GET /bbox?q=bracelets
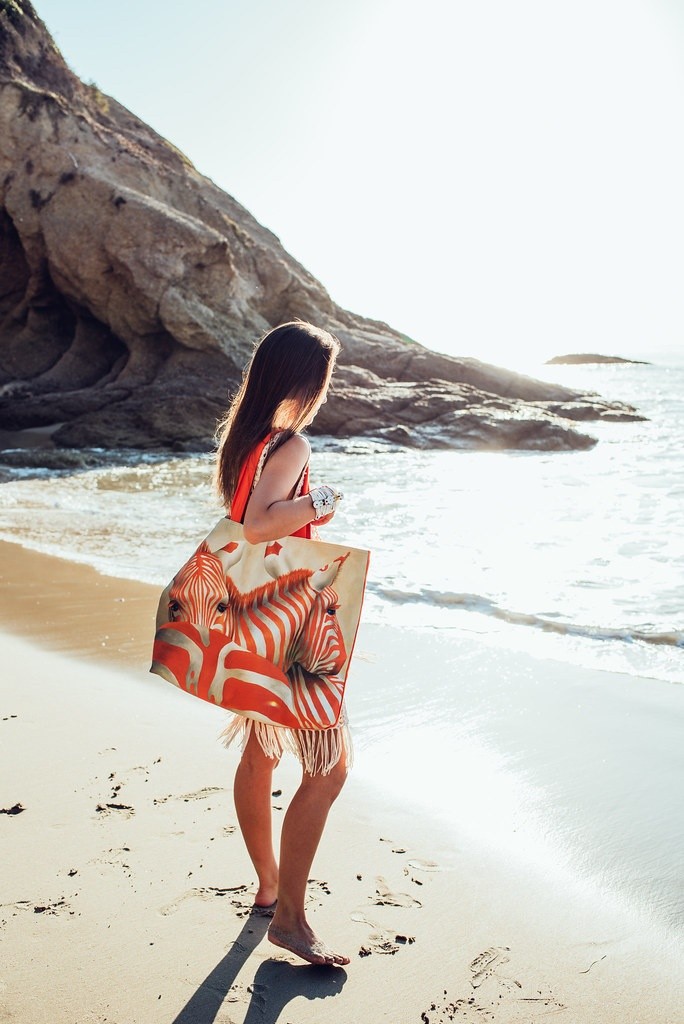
[311,484,344,523]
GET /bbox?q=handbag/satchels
[148,429,372,779]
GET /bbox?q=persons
[209,320,350,966]
